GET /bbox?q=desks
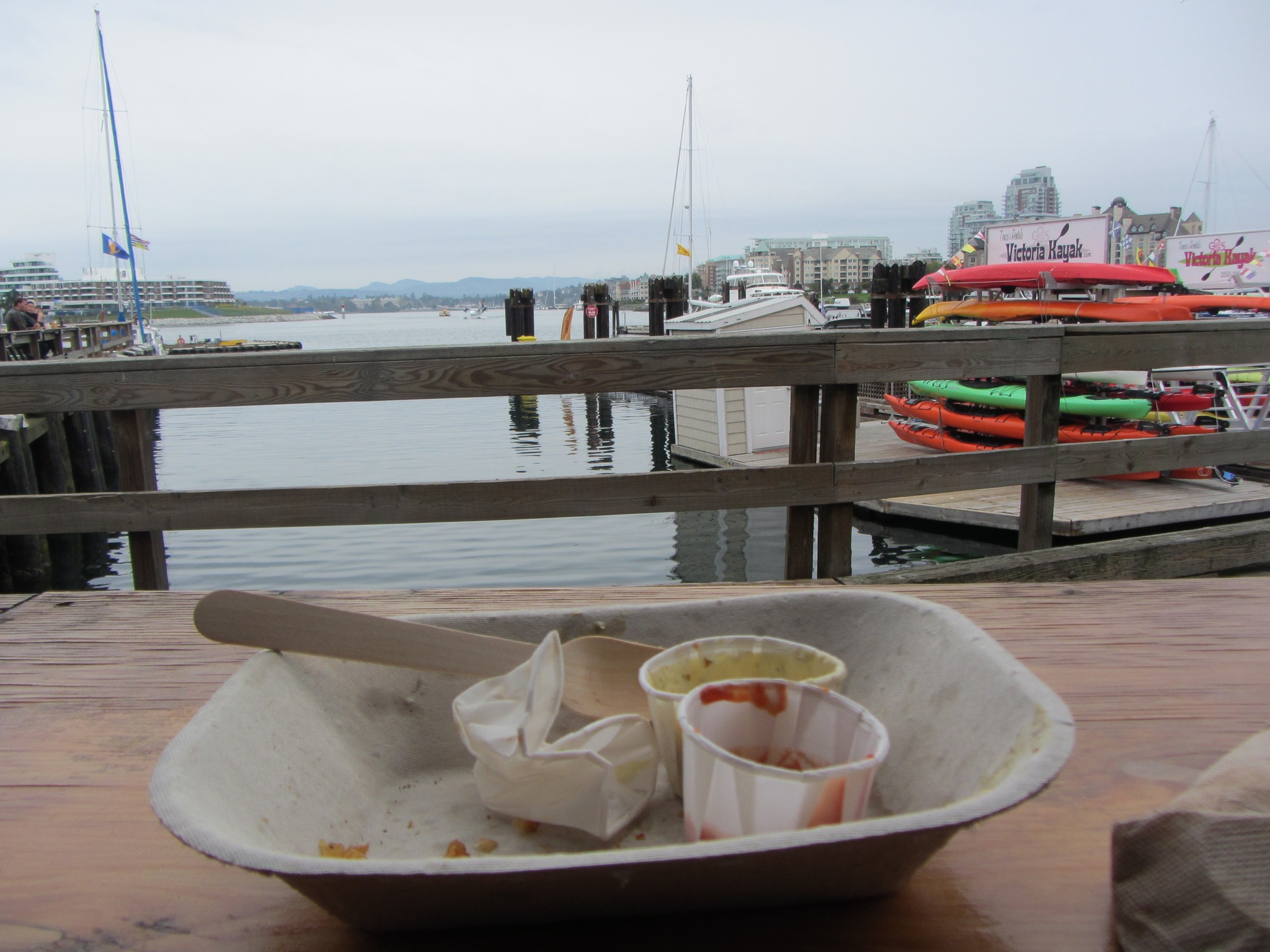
[0,577,1270,952]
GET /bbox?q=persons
[5,298,56,360]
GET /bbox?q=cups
[52,323,57,328]
[639,635,846,796]
[44,323,50,329]
[678,678,891,842]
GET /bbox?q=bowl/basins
[152,589,1076,925]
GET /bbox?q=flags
[130,234,150,250]
[676,244,690,256]
[1108,220,1166,267]
[101,233,129,261]
[936,232,990,286]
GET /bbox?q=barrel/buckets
[214,338,221,343]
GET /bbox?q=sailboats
[43,10,172,359]
[546,278,560,309]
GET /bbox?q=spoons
[193,589,669,721]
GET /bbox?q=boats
[885,264,1270,485]
[439,311,450,316]
[470,308,483,317]
[572,300,584,310]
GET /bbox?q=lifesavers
[177,338,185,345]
[142,318,149,327]
[172,342,302,354]
